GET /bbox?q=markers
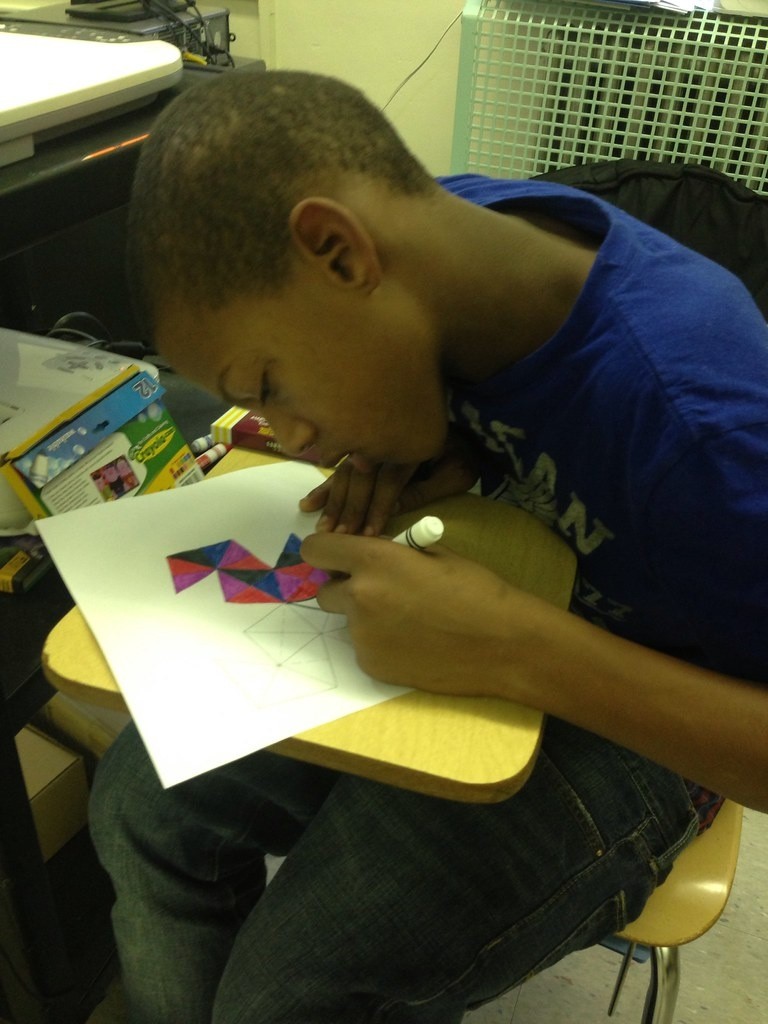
[190,430,234,471]
[382,515,446,552]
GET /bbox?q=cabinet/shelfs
[1,55,267,347]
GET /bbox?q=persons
[86,68,768,1024]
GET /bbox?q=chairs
[530,160,768,1024]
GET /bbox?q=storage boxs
[14,725,90,865]
[0,364,205,521]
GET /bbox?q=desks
[0,372,234,1024]
[42,447,578,805]
